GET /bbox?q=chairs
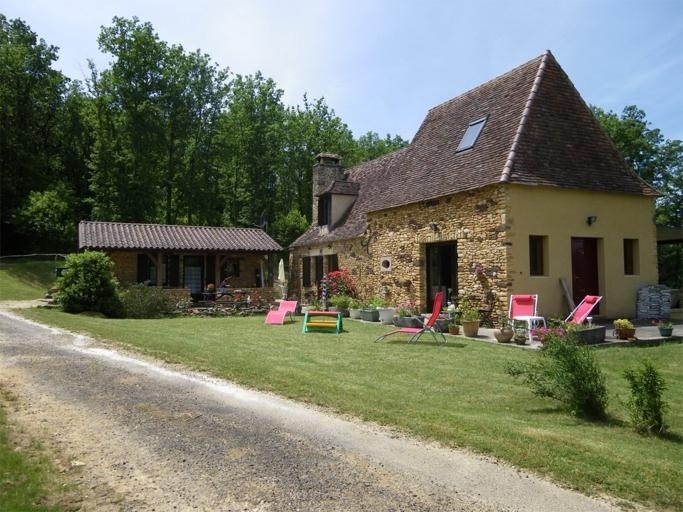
[373,291,447,345]
[265,300,299,325]
[509,294,603,343]
[478,301,496,328]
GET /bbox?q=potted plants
[327,293,481,338]
[493,315,528,345]
[612,318,637,339]
[658,320,673,336]
